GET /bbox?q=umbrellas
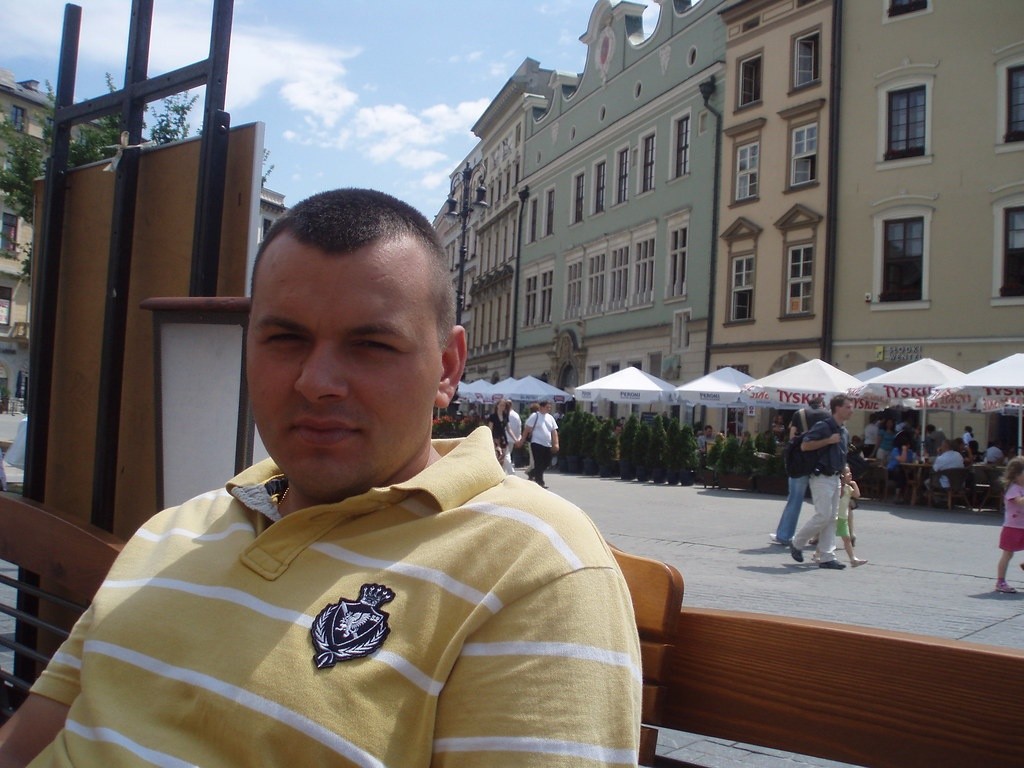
[743,358,966,462]
[573,365,757,438]
[455,375,574,403]
[935,352,1024,456]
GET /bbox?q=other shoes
[819,560,846,570]
[851,560,868,567]
[528,472,533,481]
[789,541,803,563]
[769,534,795,549]
[809,538,818,544]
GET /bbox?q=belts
[831,469,841,475]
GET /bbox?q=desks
[898,462,934,506]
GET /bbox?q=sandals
[995,583,1016,593]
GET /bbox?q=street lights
[444,160,491,329]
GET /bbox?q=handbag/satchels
[939,475,951,488]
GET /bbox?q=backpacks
[786,420,836,478]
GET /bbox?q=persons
[768,393,868,570]
[696,425,751,461]
[489,399,560,490]
[610,425,623,460]
[865,411,1024,593]
[0,185,643,768]
[769,414,785,443]
[662,411,669,432]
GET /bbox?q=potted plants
[559,411,784,494]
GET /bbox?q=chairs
[0,489,122,726]
[978,465,1006,514]
[927,468,969,511]
